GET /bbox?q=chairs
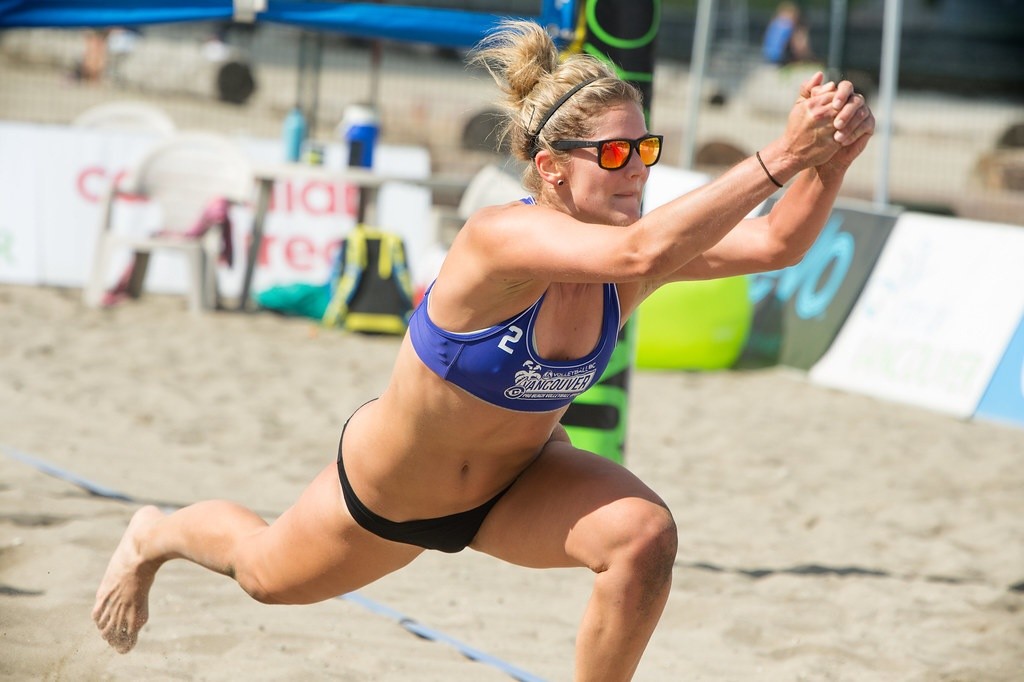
[80,129,252,309]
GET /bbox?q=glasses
[530,134,663,171]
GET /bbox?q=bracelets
[756,151,785,188]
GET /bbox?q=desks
[234,157,385,310]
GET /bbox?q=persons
[761,2,818,62]
[94,17,877,682]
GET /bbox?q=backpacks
[320,222,416,336]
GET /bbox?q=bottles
[341,100,380,166]
[282,104,305,161]
